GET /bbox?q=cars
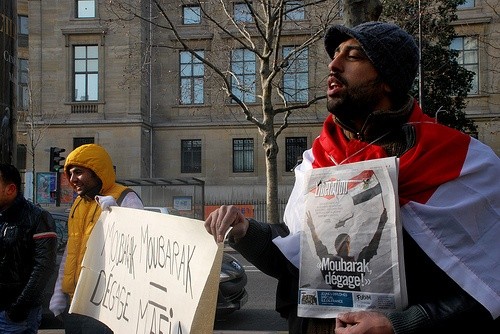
[51,212,250,325]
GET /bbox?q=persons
[204,21,500,334]
[0,163,58,334]
[305,209,388,292]
[48,143,145,334]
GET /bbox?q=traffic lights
[49,147,65,172]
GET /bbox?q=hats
[324,20,420,95]
